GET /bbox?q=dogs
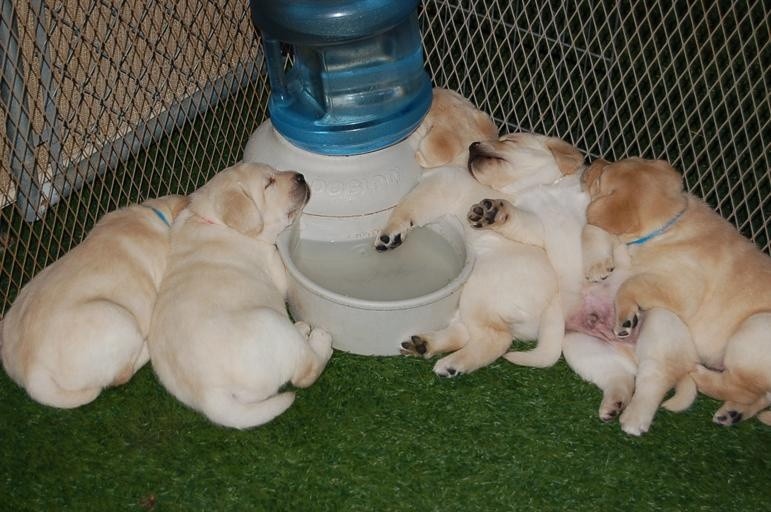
[374,87,567,380]
[148,160,334,433]
[465,130,699,438]
[581,154,771,427]
[0,192,192,411]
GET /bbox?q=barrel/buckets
[252,0,432,157]
